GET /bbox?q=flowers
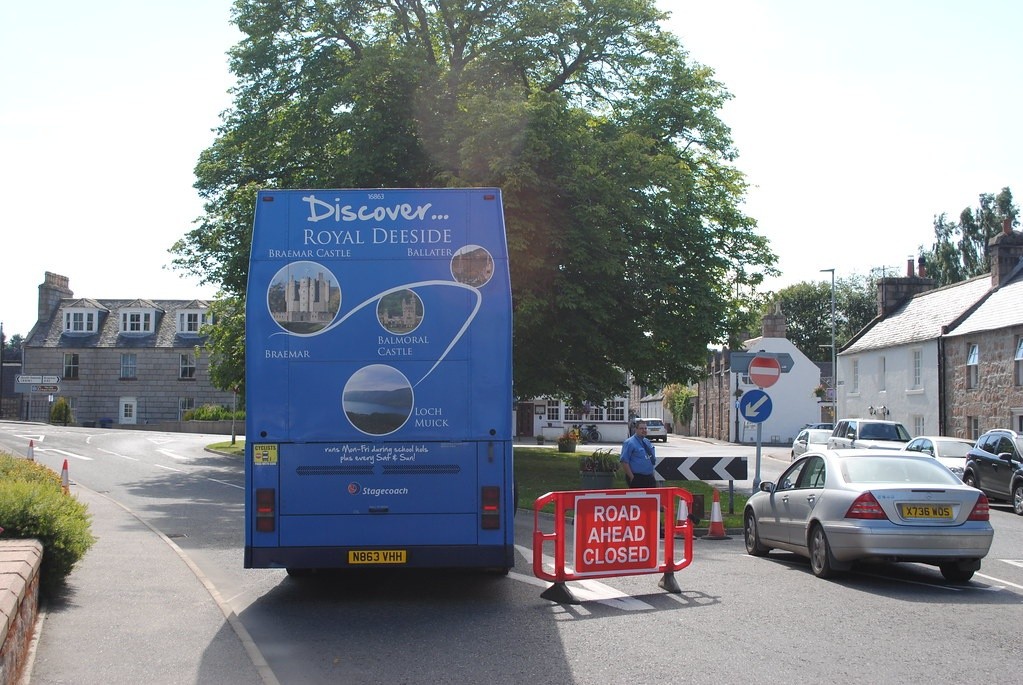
[556,428,578,446]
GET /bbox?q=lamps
[868,406,876,415]
[882,407,889,416]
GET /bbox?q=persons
[619,420,656,488]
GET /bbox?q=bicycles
[568,421,602,444]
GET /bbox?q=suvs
[961,428,1023,516]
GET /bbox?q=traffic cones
[26,440,35,462]
[673,497,698,540]
[59,459,70,495]
[700,487,733,540]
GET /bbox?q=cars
[790,429,834,463]
[899,436,979,483]
[743,449,994,582]
[801,423,836,436]
[633,418,667,442]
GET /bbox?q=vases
[558,442,576,452]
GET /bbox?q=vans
[827,418,914,455]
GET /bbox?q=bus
[241,184,518,580]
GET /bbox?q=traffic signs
[31,385,60,393]
[16,375,42,384]
[41,376,62,384]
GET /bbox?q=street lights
[820,268,838,430]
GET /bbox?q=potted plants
[581,449,621,491]
[537,435,544,445]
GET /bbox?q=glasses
[640,428,647,430]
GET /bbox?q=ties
[642,440,655,465]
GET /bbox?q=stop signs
[748,357,781,389]
